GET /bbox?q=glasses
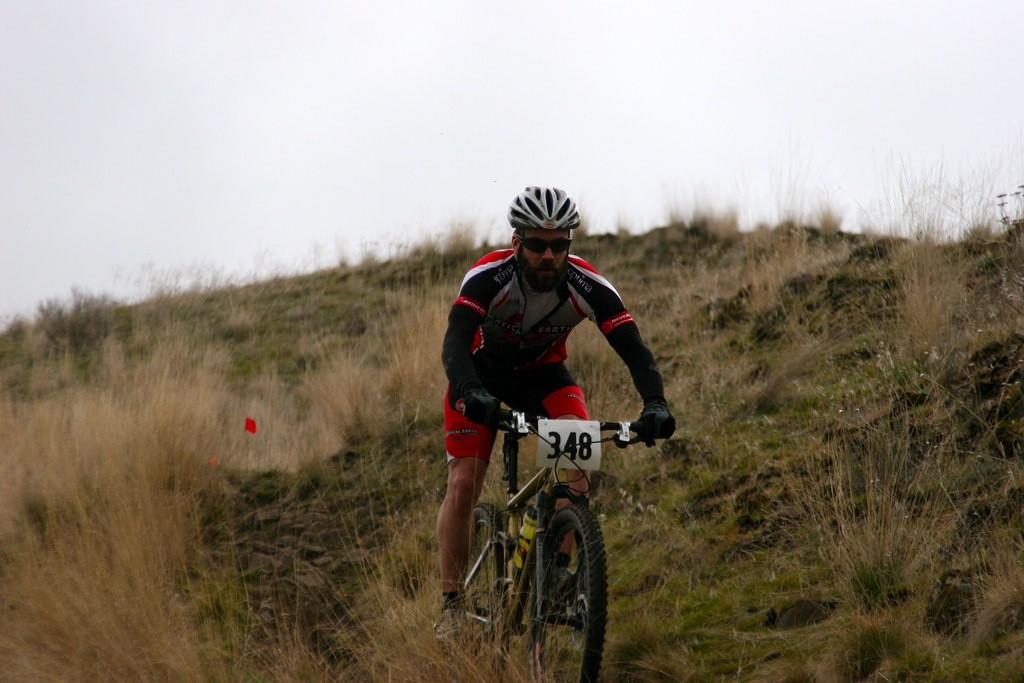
[513,232,572,254]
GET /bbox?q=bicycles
[464,397,651,683]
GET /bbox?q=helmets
[507,187,582,230]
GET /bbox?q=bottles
[513,504,538,569]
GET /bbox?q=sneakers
[554,566,574,595]
[434,606,463,641]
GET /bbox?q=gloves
[634,402,676,447]
[462,388,501,430]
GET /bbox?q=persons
[434,186,676,639]
